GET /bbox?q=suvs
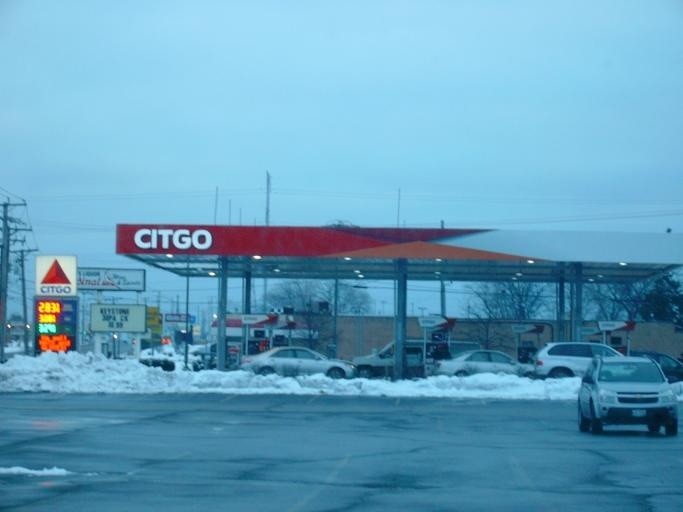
[576,355,678,438]
[532,342,636,381]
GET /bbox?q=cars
[626,350,682,383]
[241,345,357,381]
[434,349,536,382]
[136,348,175,372]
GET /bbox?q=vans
[353,339,481,381]
